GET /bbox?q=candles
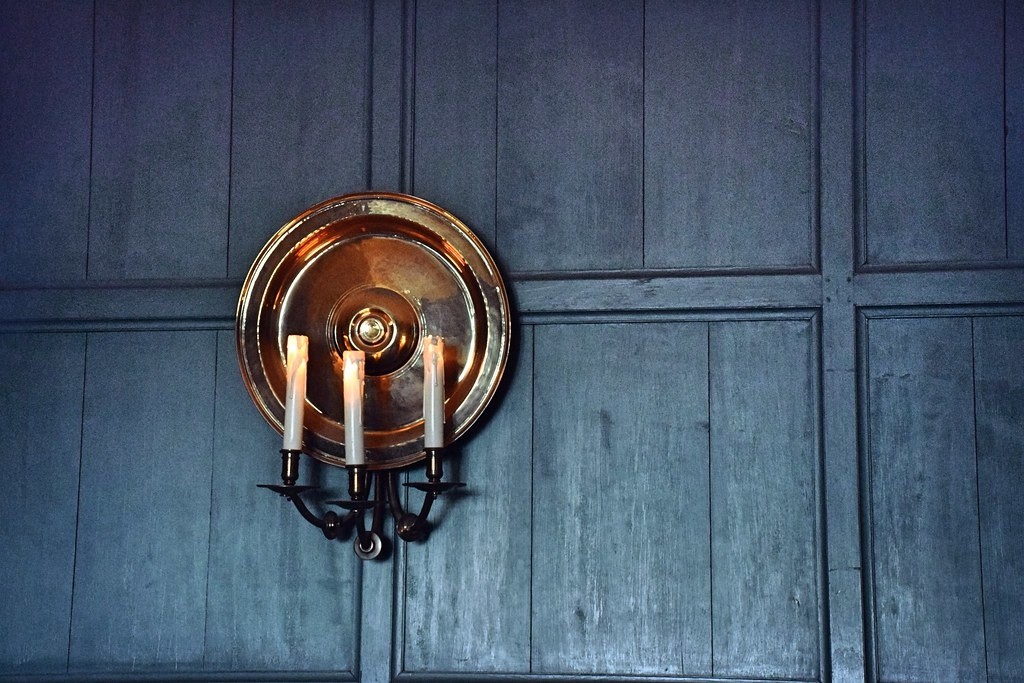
[423,334,444,447]
[283,335,309,450]
[343,351,365,465]
